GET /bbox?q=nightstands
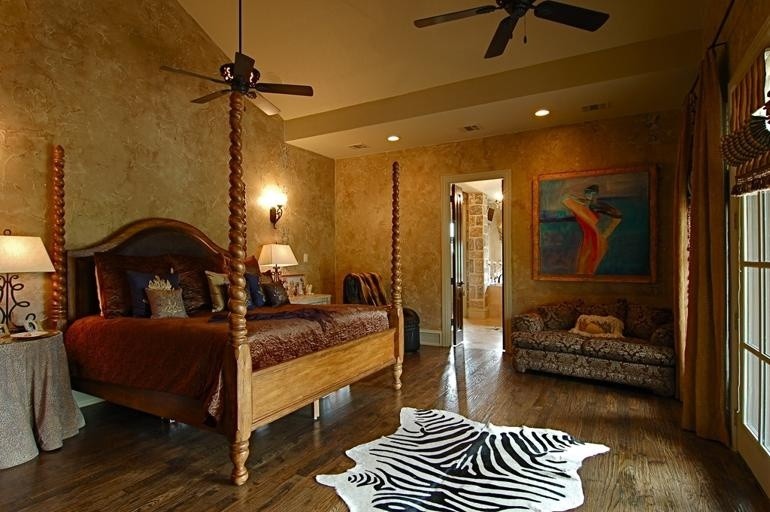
[0,330,86,471]
[286,293,332,305]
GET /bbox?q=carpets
[314,405,611,512]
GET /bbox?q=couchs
[511,299,677,395]
[342,272,419,354]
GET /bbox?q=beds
[52,91,404,486]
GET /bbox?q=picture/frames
[531,164,656,282]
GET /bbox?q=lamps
[257,244,299,281]
[1,235,56,332]
[254,183,288,229]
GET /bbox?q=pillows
[205,270,249,312]
[125,264,178,317]
[260,280,291,306]
[94,251,183,317]
[144,287,188,319]
[223,262,260,306]
[218,282,253,312]
[245,270,274,307]
[162,253,222,311]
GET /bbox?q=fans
[414,0,610,58]
[160,0,314,105]
